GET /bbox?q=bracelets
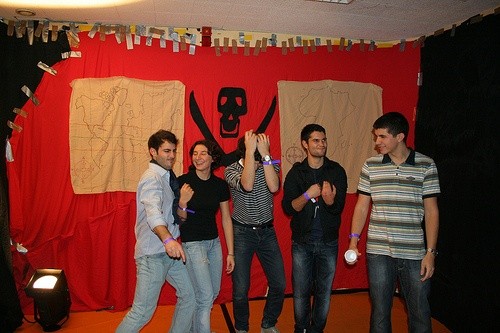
[228,254,235,256]
[303,192,311,201]
[177,203,195,213]
[263,160,280,165]
[164,238,175,244]
[426,248,438,256]
[350,233,360,237]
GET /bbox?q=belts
[232,219,274,229]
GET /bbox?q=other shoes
[235,323,246,333]
[261,326,280,333]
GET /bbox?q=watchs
[261,155,272,161]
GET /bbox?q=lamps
[23,268,72,331]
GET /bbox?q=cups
[344,249,358,265]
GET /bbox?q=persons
[224,131,286,333]
[346,112,441,333]
[281,124,348,333]
[177,139,235,333]
[115,129,196,333]
